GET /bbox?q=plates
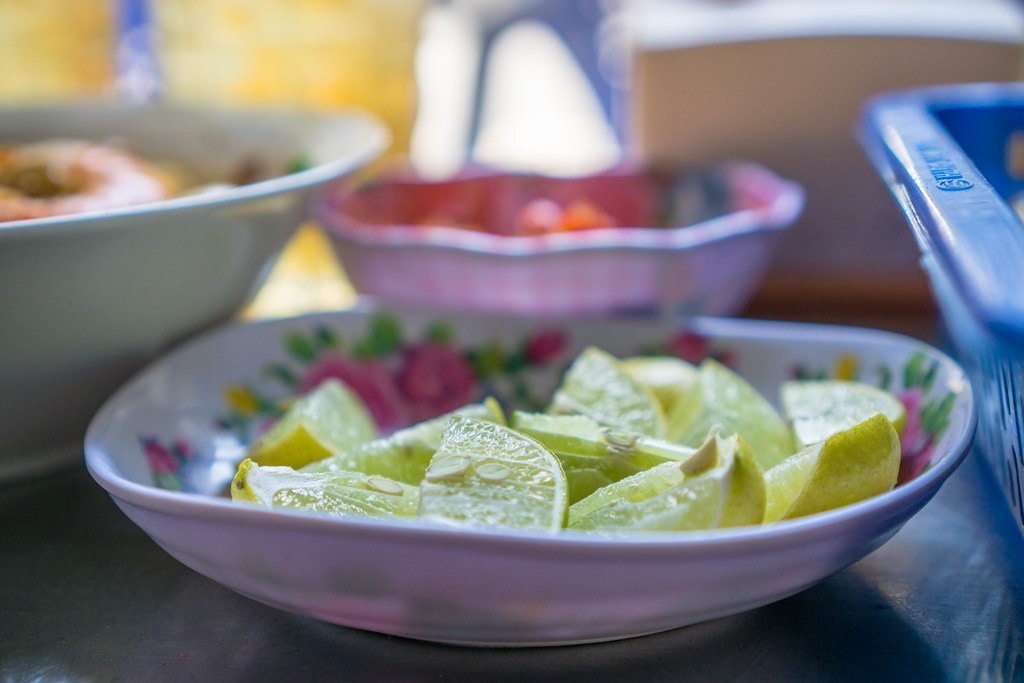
[83,314,978,648]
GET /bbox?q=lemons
[231,344,907,532]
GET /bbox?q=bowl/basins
[0,106,393,478]
[313,164,807,312]
